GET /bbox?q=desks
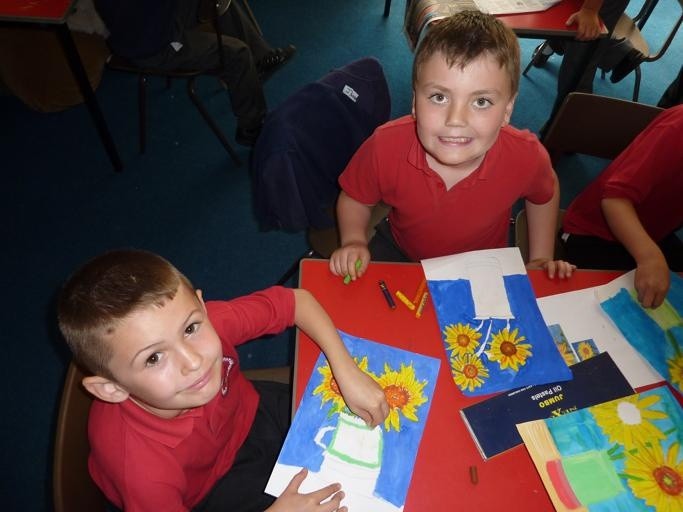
[0,0,116,151]
[291,258,683,512]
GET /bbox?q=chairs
[52,360,289,512]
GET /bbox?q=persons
[561,104,683,308]
[92,1,295,149]
[539,0,630,141]
[48,248,389,512]
[330,10,577,281]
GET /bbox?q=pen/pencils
[343,259,427,318]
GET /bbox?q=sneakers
[236,124,264,148]
[256,45,294,86]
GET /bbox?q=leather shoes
[533,44,555,67]
